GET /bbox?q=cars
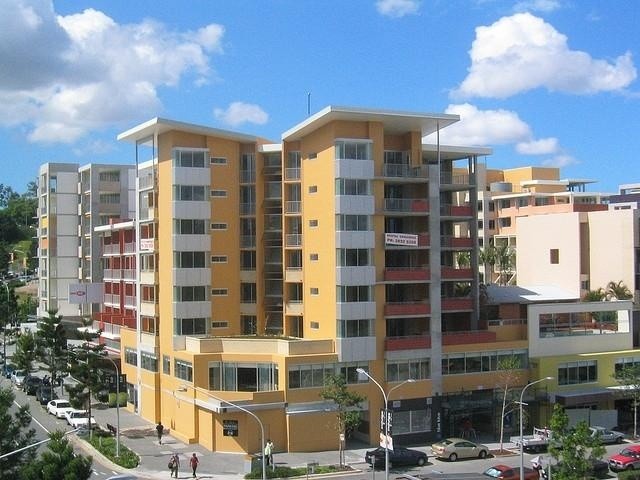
[430,437,491,462]
[365,444,429,470]
[542,457,609,480]
[572,426,625,446]
[0,353,97,430]
[607,446,640,473]
[0,269,38,288]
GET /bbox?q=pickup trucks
[483,465,539,480]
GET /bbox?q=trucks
[386,470,500,480]
[507,427,567,454]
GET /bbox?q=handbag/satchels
[168,463,173,469]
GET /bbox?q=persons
[267,439,274,465]
[189,453,199,477]
[168,452,181,478]
[156,422,165,445]
[264,443,271,465]
[463,418,473,441]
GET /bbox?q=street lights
[174,384,266,479]
[87,349,123,458]
[355,366,416,479]
[519,376,556,480]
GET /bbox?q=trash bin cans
[244,453,268,474]
